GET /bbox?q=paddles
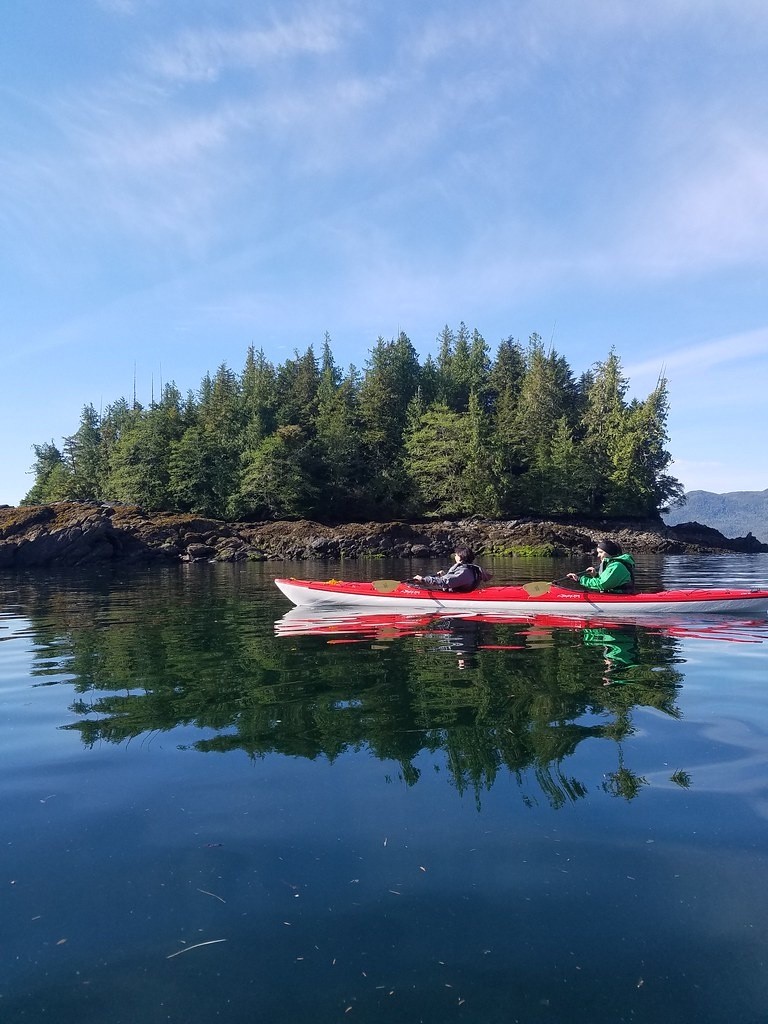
[523,566,600,598]
[371,573,439,593]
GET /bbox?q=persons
[568,538,633,593]
[416,619,480,669]
[413,548,491,592]
[570,629,642,689]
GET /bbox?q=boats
[273,576,768,617]
